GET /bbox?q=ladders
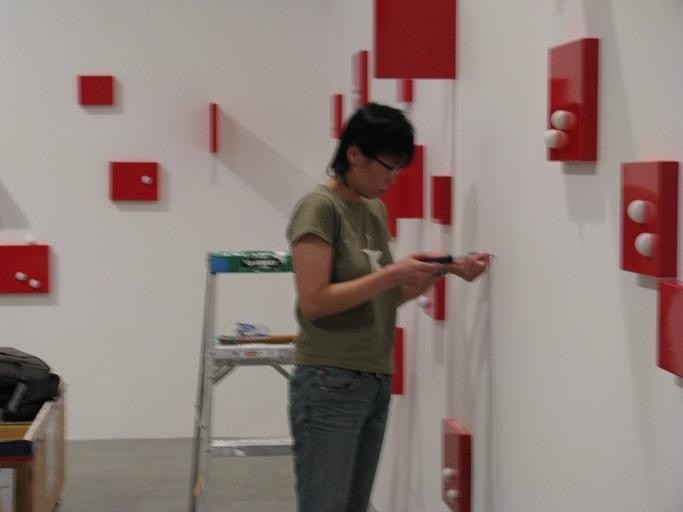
[190,251,297,512]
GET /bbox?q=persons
[285,101,491,512]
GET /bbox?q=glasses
[374,157,401,178]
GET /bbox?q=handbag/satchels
[0,346,59,420]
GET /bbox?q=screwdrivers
[434,254,484,264]
